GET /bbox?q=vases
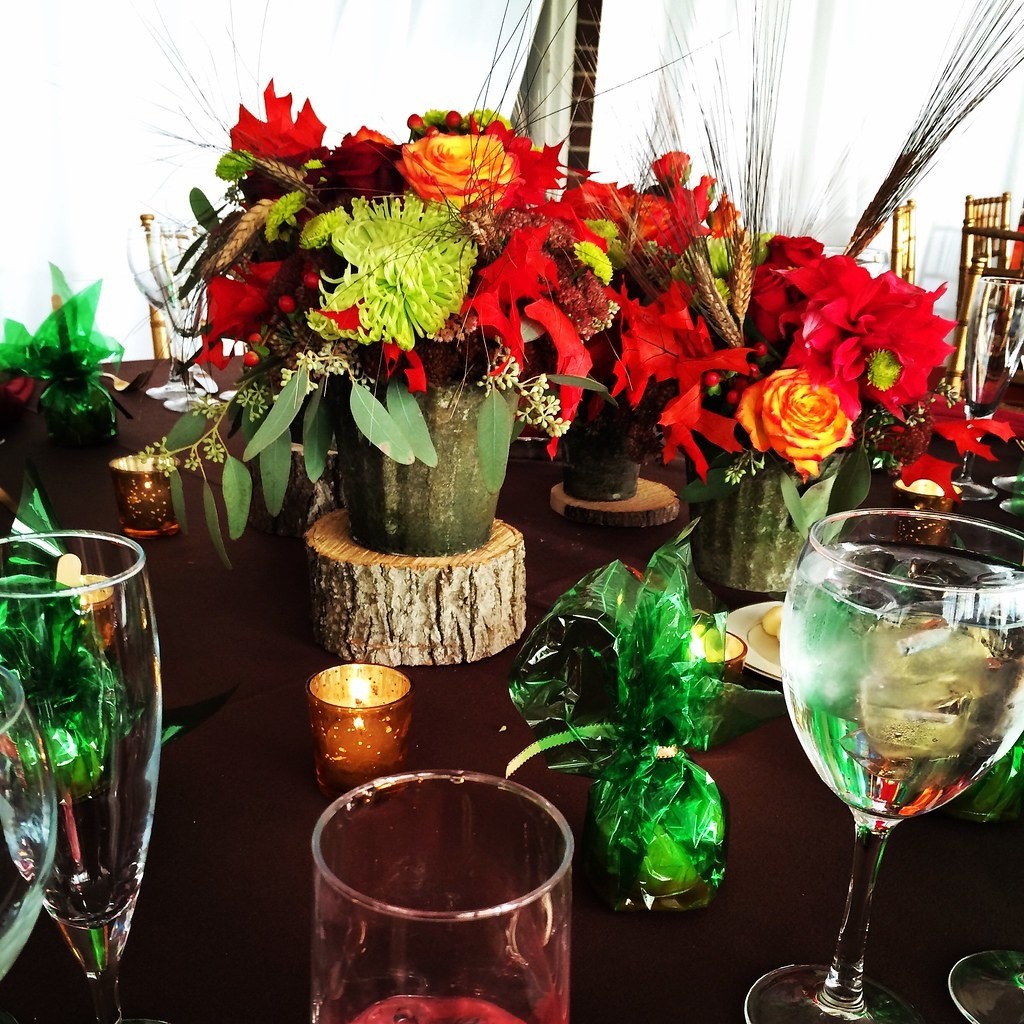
[327,362,524,560]
[689,443,853,596]
[559,369,678,501]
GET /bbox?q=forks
[101,369,153,394]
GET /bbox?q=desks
[0,357,1023,1024]
[822,242,884,276]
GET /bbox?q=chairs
[889,193,916,284]
[140,212,214,358]
[948,191,1022,402]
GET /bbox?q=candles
[896,476,962,548]
[77,573,118,649]
[686,620,747,686]
[111,449,178,538]
[306,664,411,789]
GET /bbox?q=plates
[728,601,781,684]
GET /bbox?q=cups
[0,665,58,990]
[310,771,570,1023]
[77,574,117,651]
[693,625,746,684]
[110,455,182,537]
[304,664,415,800]
[894,479,960,550]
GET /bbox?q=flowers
[124,79,1024,584]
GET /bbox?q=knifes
[188,364,218,394]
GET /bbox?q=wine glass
[158,222,220,413]
[743,508,1024,1024]
[0,530,171,1024]
[951,277,1024,517]
[948,950,1024,1024]
[220,342,249,401]
[128,224,207,401]
